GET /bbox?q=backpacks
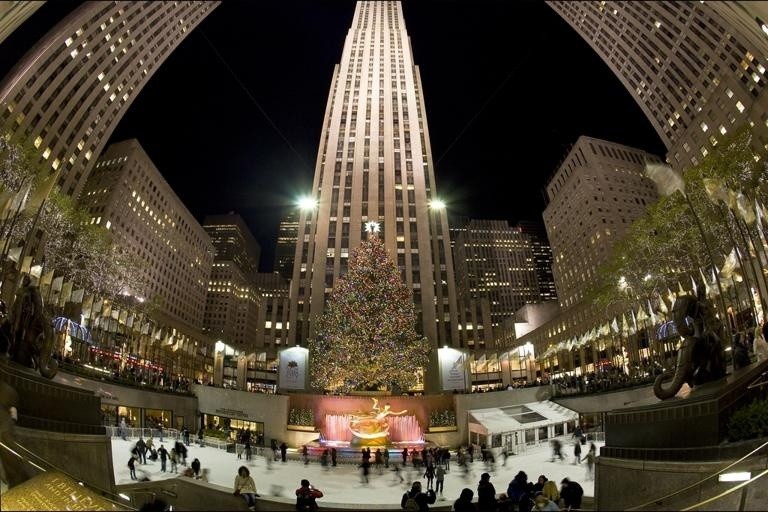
[405,491,421,512]
[542,481,560,501]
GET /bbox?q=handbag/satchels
[234,489,240,497]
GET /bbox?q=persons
[214,381,338,466]
[545,423,605,472]
[234,466,260,510]
[734,333,752,369]
[401,482,436,511]
[455,437,518,473]
[452,362,659,397]
[763,316,768,341]
[359,447,451,494]
[295,479,323,511]
[753,324,768,361]
[454,471,583,511]
[106,362,214,511]
[65,354,83,368]
